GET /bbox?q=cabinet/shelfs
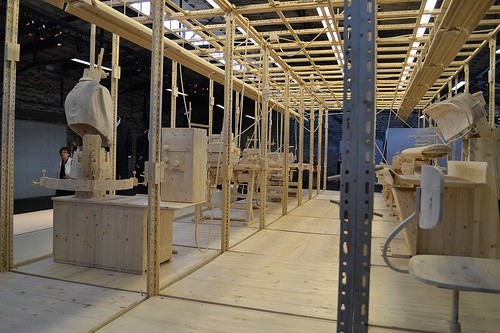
[52,194,206,274]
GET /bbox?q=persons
[57,146,71,195]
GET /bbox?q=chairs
[382,165,500,333]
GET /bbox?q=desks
[265,163,310,202]
[192,164,260,226]
[390,175,482,258]
